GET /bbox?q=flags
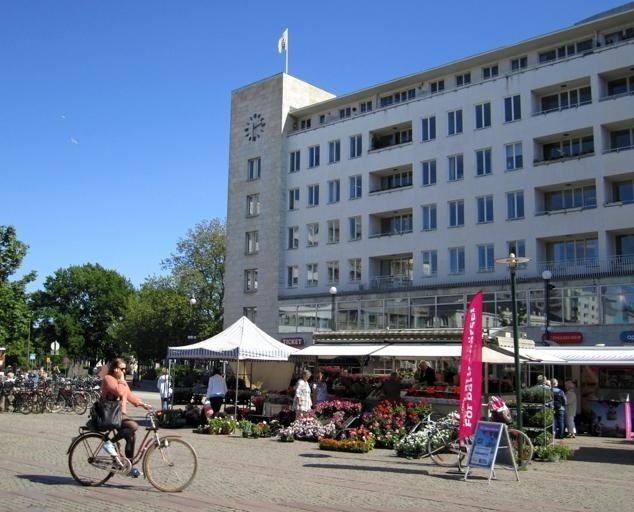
[277,30,286,54]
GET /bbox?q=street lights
[188,290,197,334]
[542,269,552,325]
[329,286,337,330]
[494,251,532,470]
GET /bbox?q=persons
[414,359,437,385]
[291,369,312,418]
[534,374,550,389]
[564,381,576,438]
[156,367,174,411]
[383,372,412,403]
[550,377,567,438]
[89,356,155,479]
[312,371,335,402]
[206,366,227,418]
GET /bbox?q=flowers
[155,411,163,426]
[198,366,521,459]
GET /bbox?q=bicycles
[425,393,534,468]
[2,373,102,415]
[66,403,197,492]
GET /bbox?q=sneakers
[102,440,118,456]
[127,472,143,477]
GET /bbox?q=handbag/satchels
[90,400,122,428]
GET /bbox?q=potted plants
[163,389,206,424]
[519,388,573,466]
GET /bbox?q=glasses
[118,367,127,371]
